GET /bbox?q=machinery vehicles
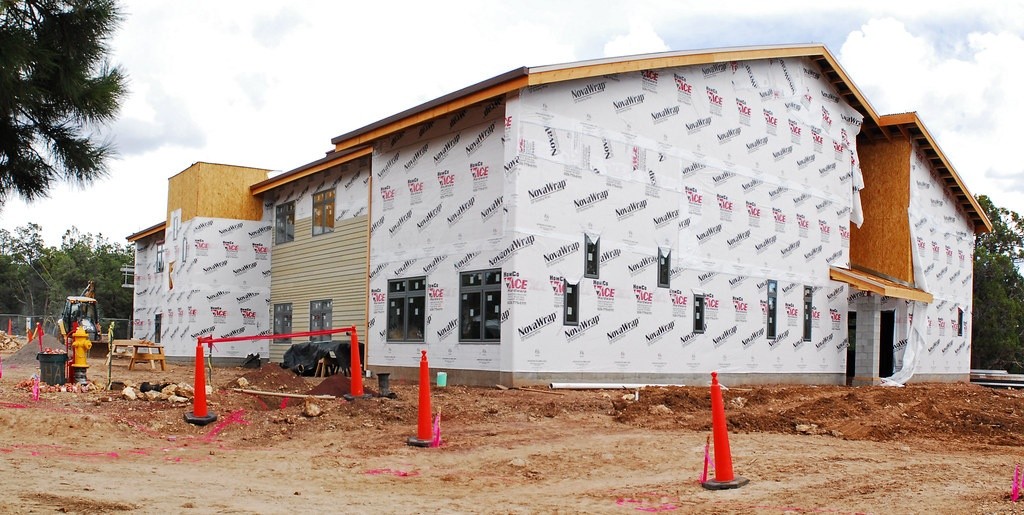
[56,280,110,359]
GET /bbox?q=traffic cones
[701,372,749,489]
[185,342,218,424]
[406,351,444,447]
[341,326,373,399]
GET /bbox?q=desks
[103,340,166,371]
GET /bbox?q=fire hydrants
[71,326,92,368]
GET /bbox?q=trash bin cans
[36,351,68,387]
